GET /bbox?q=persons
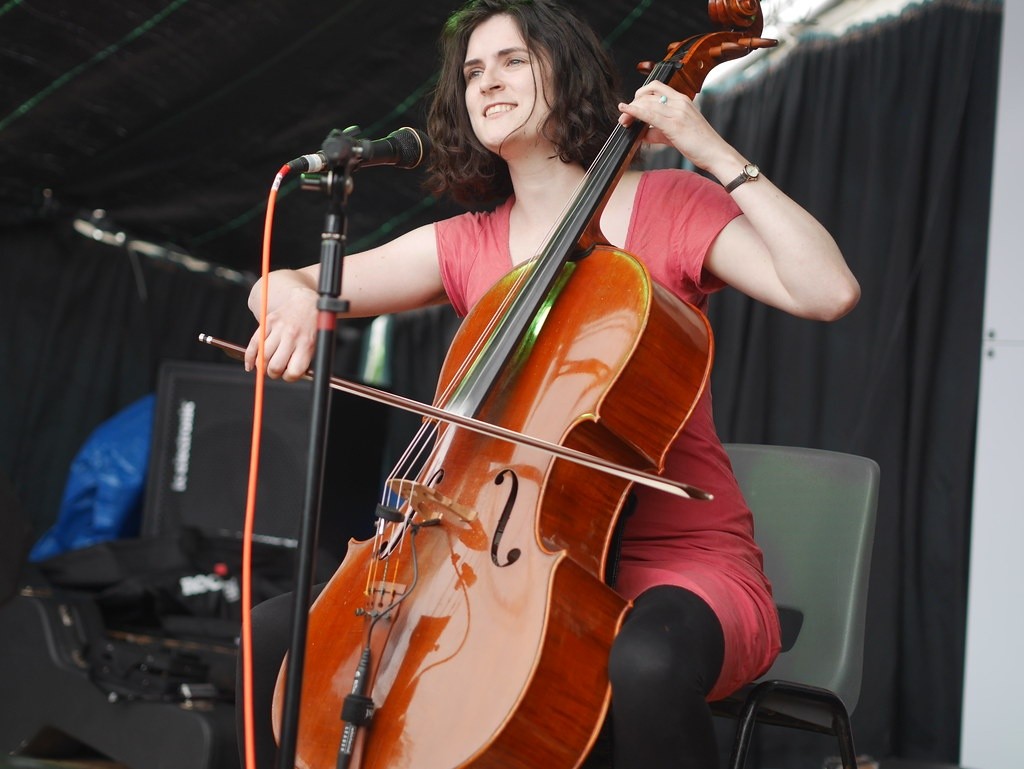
[246,0,862,769]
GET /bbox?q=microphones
[285,127,431,175]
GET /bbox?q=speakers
[138,358,391,617]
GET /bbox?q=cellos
[270,0,780,768]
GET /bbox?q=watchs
[725,163,760,193]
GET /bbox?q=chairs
[709,441,881,768]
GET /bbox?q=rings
[660,96,667,104]
